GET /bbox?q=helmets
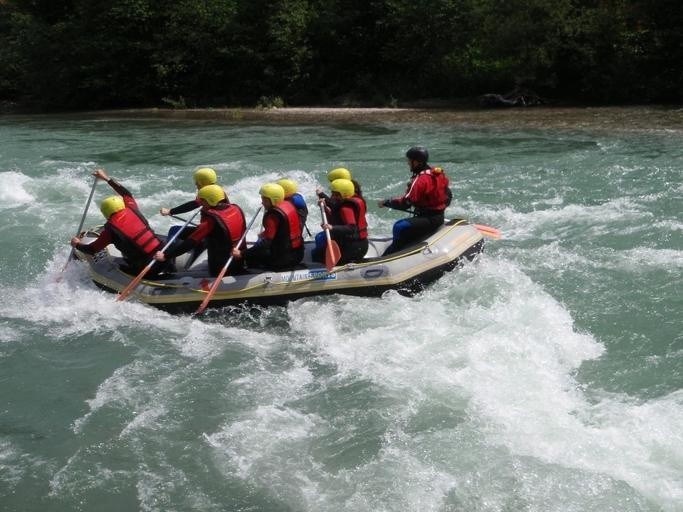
[278,179,296,198]
[199,185,225,207]
[259,183,284,207]
[406,146,428,162]
[100,196,125,220]
[327,168,352,181]
[193,168,216,188]
[331,179,355,199]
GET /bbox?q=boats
[69,217,486,317]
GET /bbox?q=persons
[69,167,170,281]
[158,167,230,274]
[314,167,366,217]
[310,177,369,266]
[150,183,247,278]
[275,179,308,235]
[230,182,305,271]
[375,145,452,258]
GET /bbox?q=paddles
[116,206,204,303]
[384,204,500,239]
[321,201,342,272]
[198,201,264,315]
